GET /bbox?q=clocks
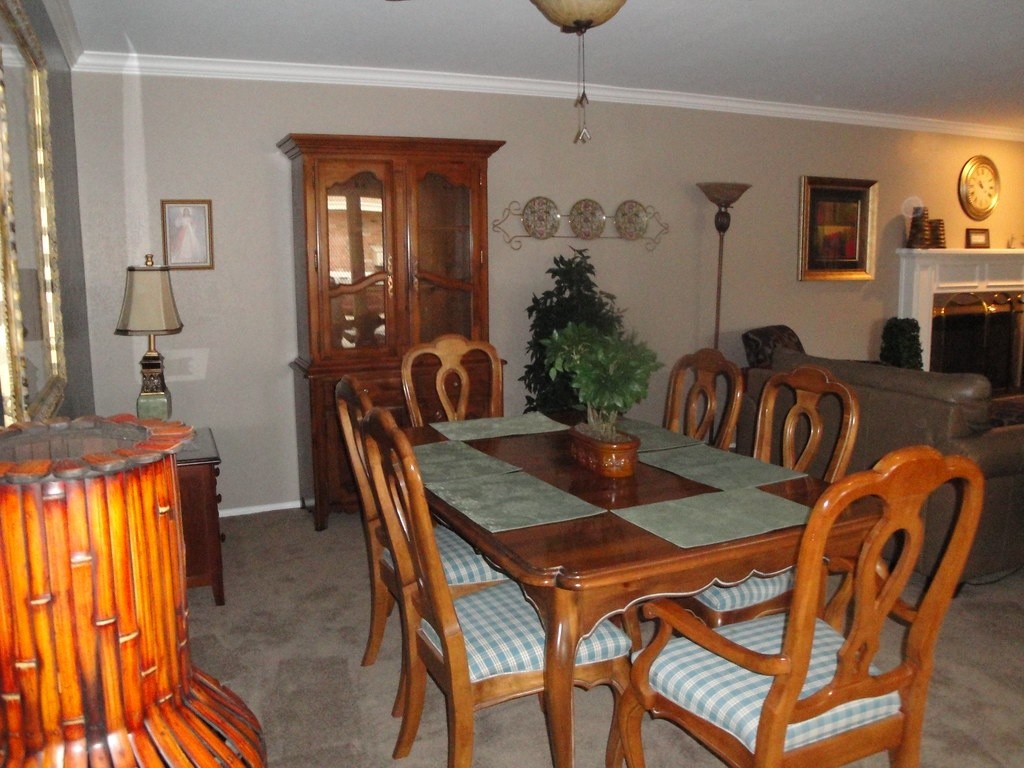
[959,155,1000,220]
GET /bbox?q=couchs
[736,346,1024,597]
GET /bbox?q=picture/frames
[797,175,878,281]
[966,229,990,248]
[161,199,215,269]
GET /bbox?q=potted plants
[540,321,665,478]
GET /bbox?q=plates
[522,197,561,240]
[614,200,648,240]
[569,199,607,240]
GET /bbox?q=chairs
[359,406,631,768]
[619,446,986,768]
[663,348,744,450]
[402,334,504,427]
[332,375,511,716]
[754,364,861,483]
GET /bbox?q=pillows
[741,325,805,368]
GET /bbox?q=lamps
[532,0,629,140]
[113,254,184,420]
[695,181,752,349]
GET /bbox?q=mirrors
[1,0,68,428]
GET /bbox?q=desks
[376,415,886,768]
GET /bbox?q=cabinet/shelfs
[174,426,225,606]
[276,133,506,530]
